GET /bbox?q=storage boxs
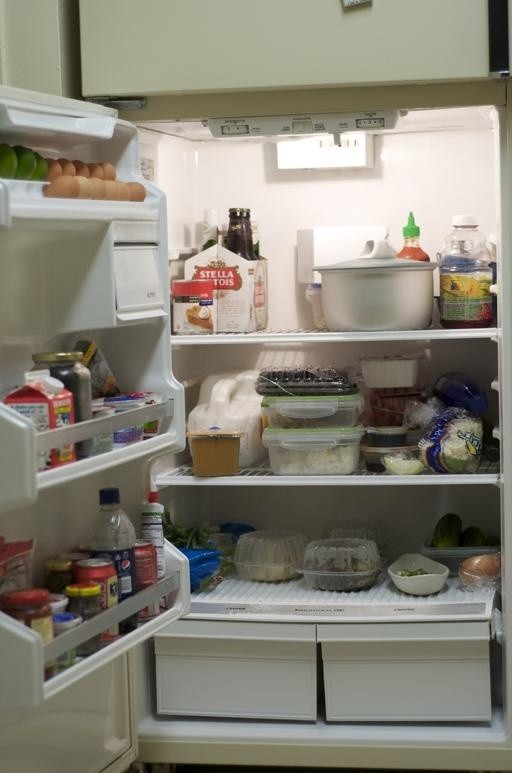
[317,623,492,723]
[154,620,318,721]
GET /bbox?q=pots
[312,238,437,332]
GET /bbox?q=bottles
[400,211,428,261]
[140,492,165,581]
[438,214,495,329]
[88,487,138,635]
[226,207,256,261]
[30,351,94,458]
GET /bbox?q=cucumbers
[428,513,500,548]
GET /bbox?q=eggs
[45,158,146,201]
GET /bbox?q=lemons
[0,143,48,180]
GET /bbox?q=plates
[386,550,451,595]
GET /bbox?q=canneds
[172,280,214,335]
[134,539,161,620]
[0,588,56,681]
[75,558,119,642]
